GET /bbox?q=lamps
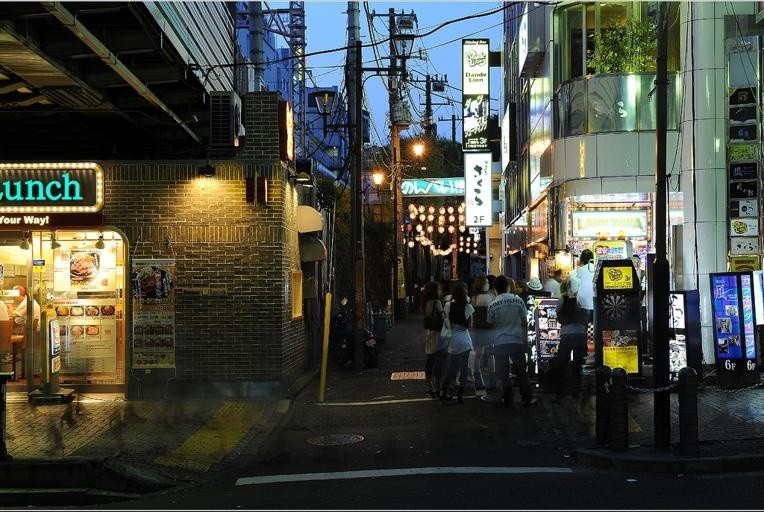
[17,228,106,253]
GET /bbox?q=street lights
[308,32,417,366]
[370,137,428,313]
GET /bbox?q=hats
[527,279,543,290]
[560,277,581,296]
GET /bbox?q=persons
[0,299,9,320]
[632,253,646,285]
[424,249,595,408]
[7,286,41,331]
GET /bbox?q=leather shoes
[424,388,464,402]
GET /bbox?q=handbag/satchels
[424,315,444,331]
[576,306,586,326]
[440,318,452,337]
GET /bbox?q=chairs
[0,319,43,379]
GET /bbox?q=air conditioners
[206,89,242,153]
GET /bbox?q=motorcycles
[330,292,383,371]
